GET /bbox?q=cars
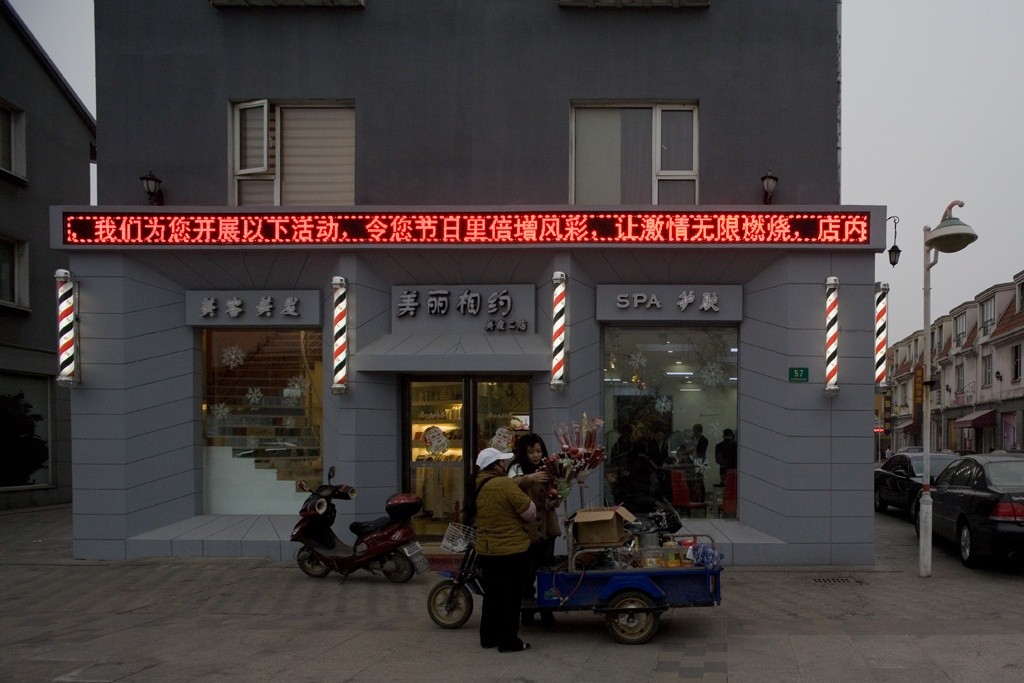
[874,451,970,527]
[915,453,1024,571]
[902,446,923,452]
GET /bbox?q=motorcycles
[290,465,432,584]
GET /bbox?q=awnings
[953,409,996,427]
[895,421,913,432]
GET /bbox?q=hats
[476,448,514,470]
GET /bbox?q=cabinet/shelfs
[410,381,491,468]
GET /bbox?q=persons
[691,424,708,501]
[886,445,892,459]
[715,429,737,485]
[474,448,536,653]
[605,424,668,514]
[505,432,562,626]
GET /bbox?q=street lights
[918,200,978,578]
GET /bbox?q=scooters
[427,521,725,646]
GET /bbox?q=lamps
[946,384,951,393]
[825,277,840,398]
[53,268,81,388]
[549,271,566,392]
[874,281,889,393]
[331,275,348,394]
[138,168,163,206]
[995,371,1002,382]
[886,216,902,269]
[761,170,778,205]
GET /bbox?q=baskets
[440,523,476,554]
[656,512,682,533]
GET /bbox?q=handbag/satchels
[655,496,680,524]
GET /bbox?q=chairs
[671,471,737,519]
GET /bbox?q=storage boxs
[569,506,637,544]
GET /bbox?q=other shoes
[542,613,555,624]
[521,614,534,627]
[499,640,530,652]
